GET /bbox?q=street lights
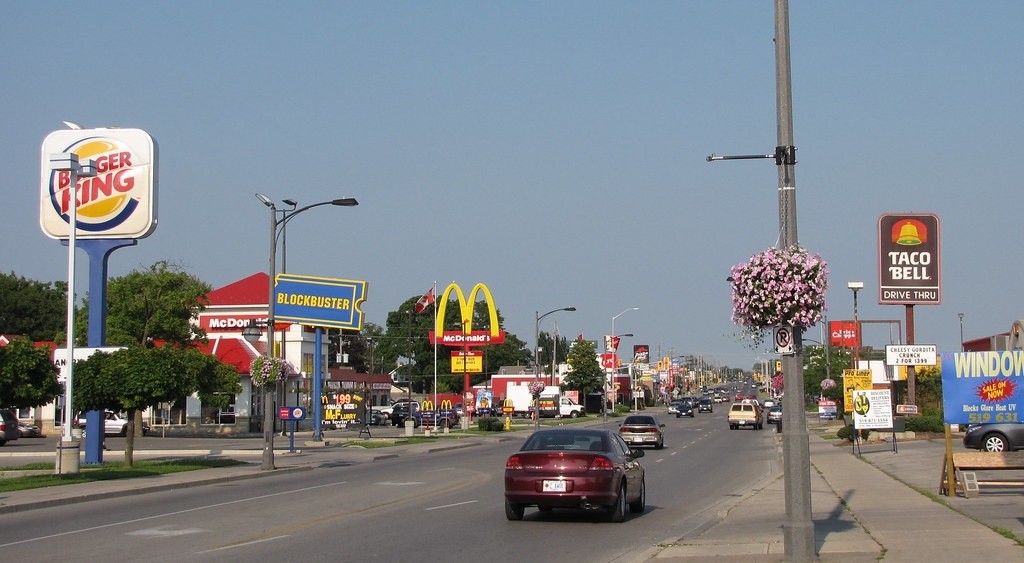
[603,307,640,422]
[47,152,98,440]
[534,307,578,430]
[958,312,965,352]
[847,281,864,370]
[243,192,359,474]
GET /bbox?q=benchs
[359,424,372,438]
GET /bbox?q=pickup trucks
[727,402,763,430]
[495,400,504,415]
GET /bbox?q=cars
[962,420,1024,453]
[619,415,666,450]
[19,422,39,438]
[764,399,783,423]
[676,403,694,418]
[668,377,774,414]
[452,404,463,417]
[366,409,386,425]
[504,427,646,522]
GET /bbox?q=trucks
[392,406,459,429]
[506,381,585,419]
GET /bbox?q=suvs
[0,409,19,446]
[380,399,421,420]
[73,408,150,437]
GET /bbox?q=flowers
[247,356,291,387]
[528,381,547,394]
[770,371,784,390]
[726,239,830,347]
[820,377,836,391]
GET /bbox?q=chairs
[589,441,603,451]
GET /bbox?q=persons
[105,412,111,420]
